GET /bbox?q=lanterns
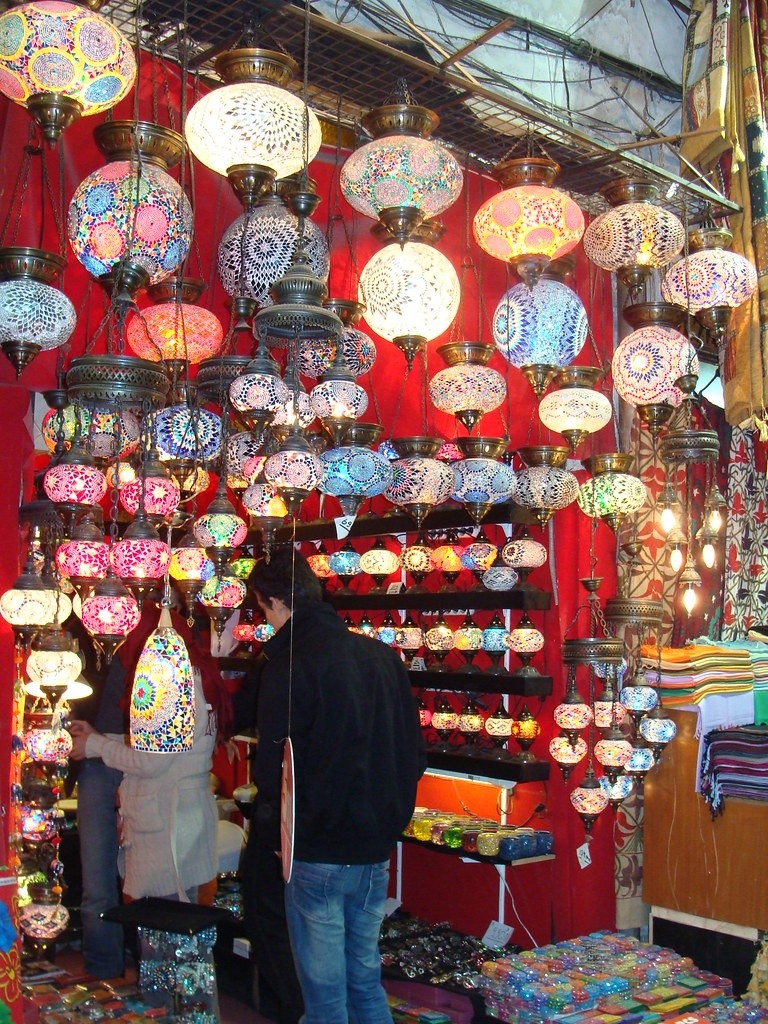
[549,677,677,833]
[2,1,757,529]
[0,558,83,948]
[232,537,547,752]
[55,513,246,664]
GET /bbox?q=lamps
[599,541,678,815]
[0,528,255,849]
[582,179,685,302]
[512,446,579,532]
[255,537,547,760]
[537,366,612,456]
[493,259,589,402]
[576,453,647,532]
[548,577,634,837]
[472,121,585,292]
[611,303,700,446]
[661,200,758,351]
[0,0,518,528]
[659,375,727,618]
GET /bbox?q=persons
[231,546,429,1024]
[65,561,245,980]
